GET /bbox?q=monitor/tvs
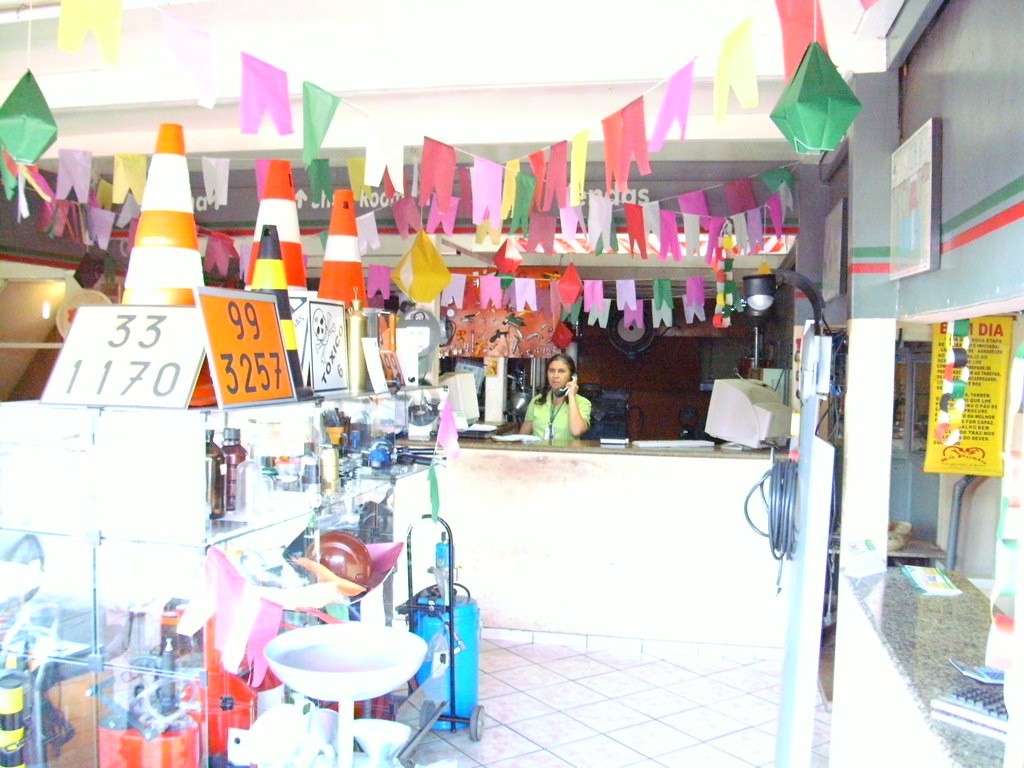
[705,379,792,451]
[439,372,480,426]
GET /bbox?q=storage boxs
[832,363,932,427]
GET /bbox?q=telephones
[554,372,578,398]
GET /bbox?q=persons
[518,353,592,440]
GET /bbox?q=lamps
[742,274,777,311]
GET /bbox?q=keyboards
[930,685,1010,732]
[632,439,714,448]
[468,423,497,431]
